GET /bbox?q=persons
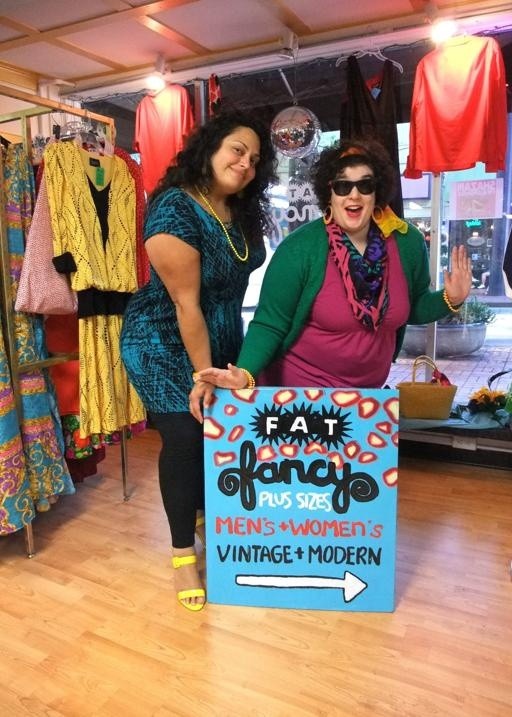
[117,107,281,612]
[193,138,474,392]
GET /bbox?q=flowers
[467,386,512,429]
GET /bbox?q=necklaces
[191,183,249,263]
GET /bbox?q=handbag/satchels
[395,356,457,419]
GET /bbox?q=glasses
[330,179,375,195]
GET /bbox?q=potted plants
[402,295,496,360]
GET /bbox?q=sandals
[172,555,206,610]
[196,517,206,551]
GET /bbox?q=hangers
[48,103,117,161]
[334,37,404,74]
[429,19,482,48]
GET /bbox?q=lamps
[282,30,299,59]
[145,53,167,91]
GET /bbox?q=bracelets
[236,363,258,393]
[443,289,462,312]
[192,372,201,384]
